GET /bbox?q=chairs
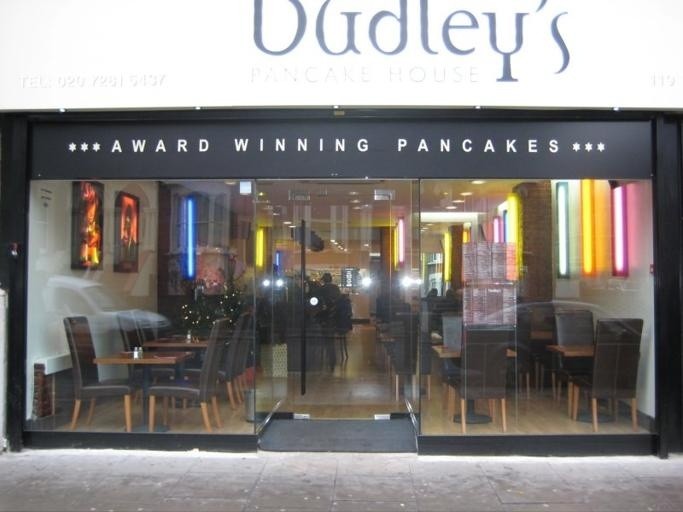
[374,294,643,434]
[64,310,250,432]
[325,292,353,363]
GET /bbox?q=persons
[376,280,462,396]
[119,205,137,263]
[280,272,342,372]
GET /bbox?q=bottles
[133,346,143,360]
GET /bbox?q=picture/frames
[111,191,139,274]
[71,182,103,269]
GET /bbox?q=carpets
[262,418,413,453]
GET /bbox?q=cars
[445,298,644,386]
[43,274,171,365]
